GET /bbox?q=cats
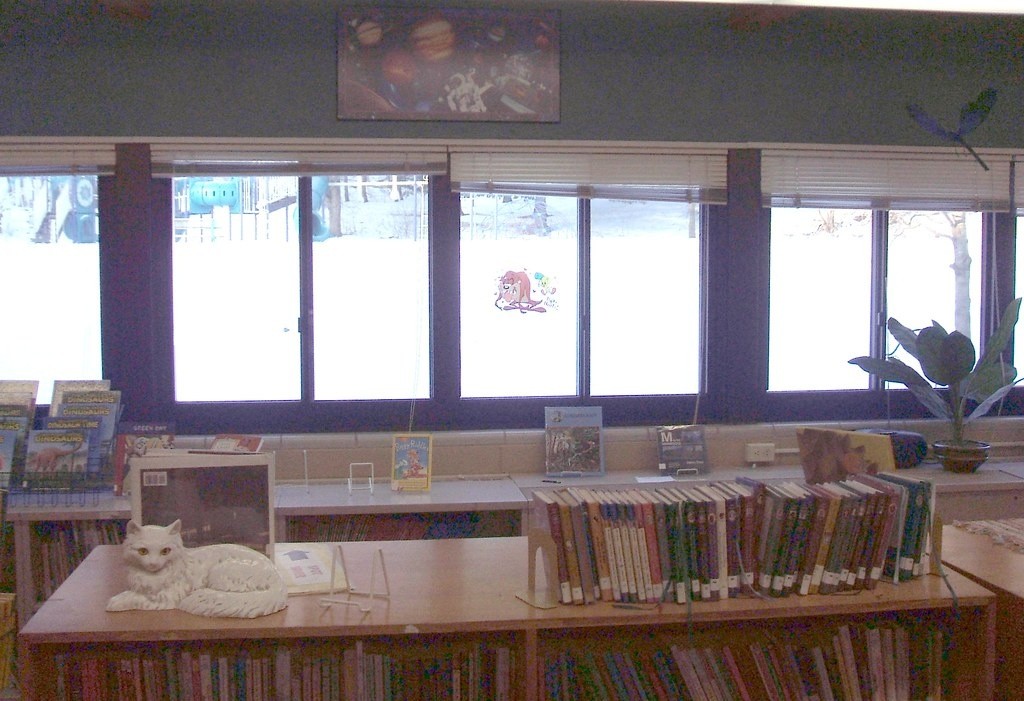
[107,519,288,619]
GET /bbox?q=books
[285,510,521,543]
[390,433,433,492]
[544,405,607,478]
[0,379,266,499]
[127,450,276,575]
[34,520,128,602]
[532,471,937,605]
[655,425,708,477]
[795,426,896,485]
[538,623,988,701]
[55,639,527,700]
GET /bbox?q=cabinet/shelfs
[1,453,1024,701]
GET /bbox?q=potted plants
[843,294,1021,472]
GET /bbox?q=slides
[292,175,332,242]
